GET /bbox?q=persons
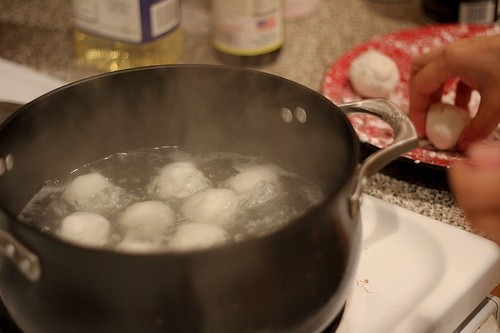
[409,31,500,241]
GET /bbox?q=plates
[321,24,500,168]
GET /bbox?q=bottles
[70,0,185,72]
[210,0,286,67]
[420,0,498,26]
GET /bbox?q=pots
[0,64,419,333]
